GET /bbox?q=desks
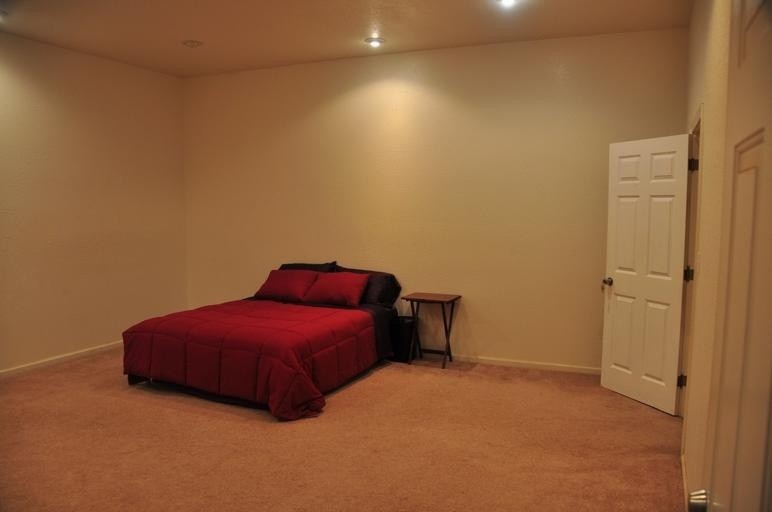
[401,292,463,370]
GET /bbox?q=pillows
[254,268,370,309]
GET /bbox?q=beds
[119,263,402,421]
